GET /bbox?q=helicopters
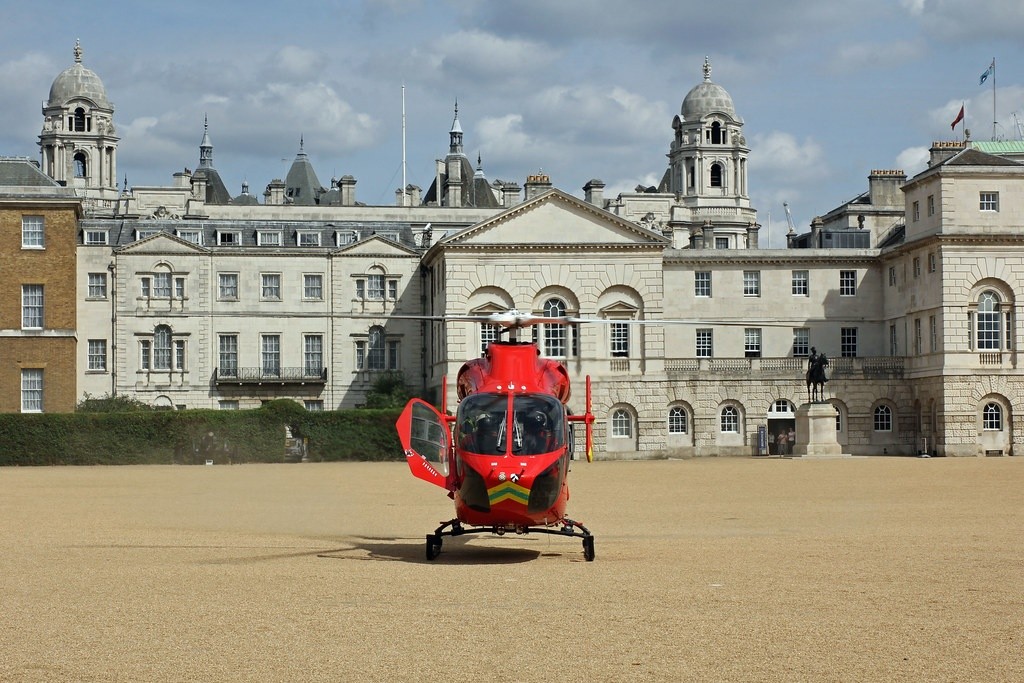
[108,307,812,560]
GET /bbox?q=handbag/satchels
[777,442,780,445]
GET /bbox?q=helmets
[475,414,496,432]
[523,410,547,435]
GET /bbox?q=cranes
[783,203,798,234]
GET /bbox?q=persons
[519,411,558,453]
[461,413,497,453]
[768,427,797,458]
[808,346,829,387]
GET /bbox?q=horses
[806,353,829,403]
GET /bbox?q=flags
[951,107,964,132]
[980,64,993,85]
